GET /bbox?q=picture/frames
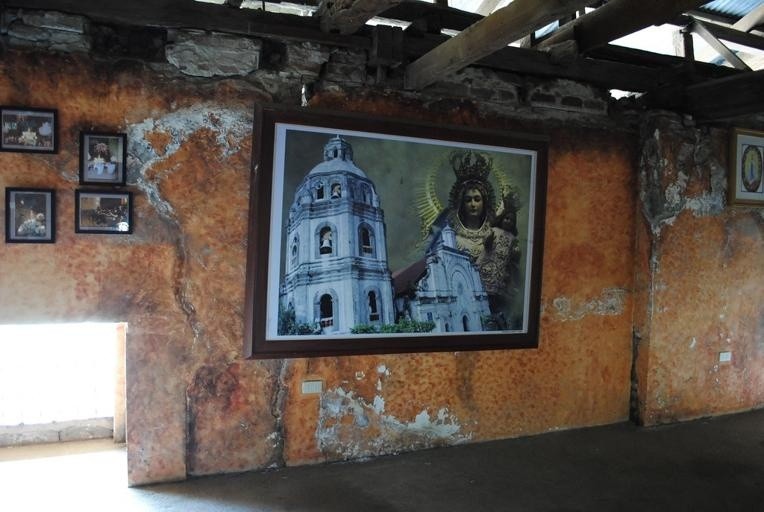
[729,126,764,207]
[240,102,549,362]
[1,107,133,243]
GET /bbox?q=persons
[422,177,523,313]
[491,207,520,237]
